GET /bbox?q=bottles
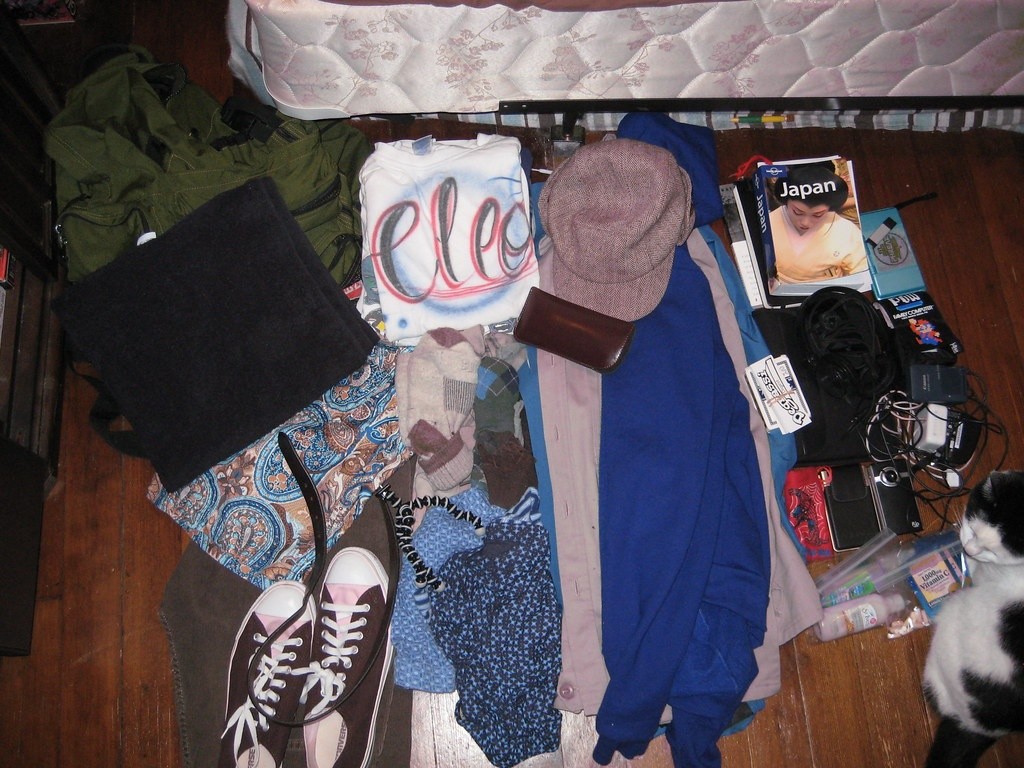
[813,592,905,642]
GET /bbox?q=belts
[247,432,401,726]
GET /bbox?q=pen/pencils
[730,115,794,124]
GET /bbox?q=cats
[919,470,1024,767]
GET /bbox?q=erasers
[866,217,898,247]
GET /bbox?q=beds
[226,0,1024,133]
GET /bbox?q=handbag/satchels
[48,179,382,494]
[44,56,363,289]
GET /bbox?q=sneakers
[301,547,395,768]
[219,581,317,768]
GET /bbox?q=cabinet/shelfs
[0,0,135,660]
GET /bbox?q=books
[730,155,872,308]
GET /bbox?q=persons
[769,163,866,280]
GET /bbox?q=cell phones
[868,458,923,534]
[819,465,882,552]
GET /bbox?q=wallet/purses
[781,466,837,562]
[516,288,638,374]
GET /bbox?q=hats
[541,136,694,321]
